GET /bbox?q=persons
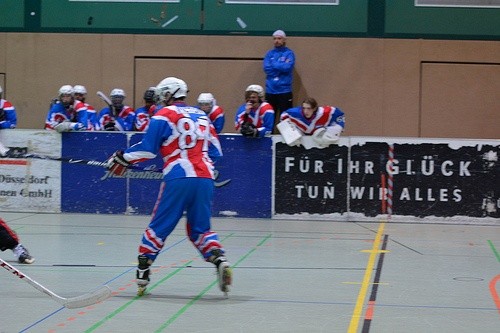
[262,30,295,134]
[277,99,345,147]
[132,85,164,131]
[44,85,99,131]
[0,86,17,129]
[0,217,36,264]
[235,84,275,137]
[195,92,225,134]
[97,88,136,131]
[107,75,234,298]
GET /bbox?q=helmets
[155,76,187,102]
[246,84,264,98]
[73,85,87,95]
[58,85,74,98]
[197,92,214,103]
[110,89,127,96]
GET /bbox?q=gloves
[240,121,259,137]
[104,150,131,177]
[103,122,117,131]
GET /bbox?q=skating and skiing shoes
[12,244,35,264]
[136,254,152,296]
[208,249,232,299]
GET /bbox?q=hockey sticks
[0,143,163,173]
[96,91,116,117]
[0,257,112,309]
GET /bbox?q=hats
[273,29,286,38]
